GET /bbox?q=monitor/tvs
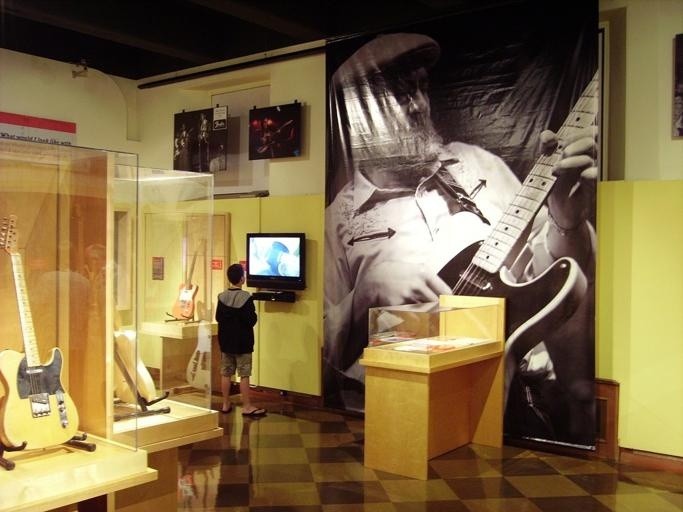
[244,232,305,289]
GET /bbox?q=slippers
[242,409,267,416]
[221,405,232,413]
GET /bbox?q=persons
[215,412,263,512]
[215,263,268,417]
[326,50,597,447]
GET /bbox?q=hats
[329,33,440,91]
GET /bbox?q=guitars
[172,251,198,320]
[0,214,80,451]
[438,70,599,414]
[114,334,156,404]
